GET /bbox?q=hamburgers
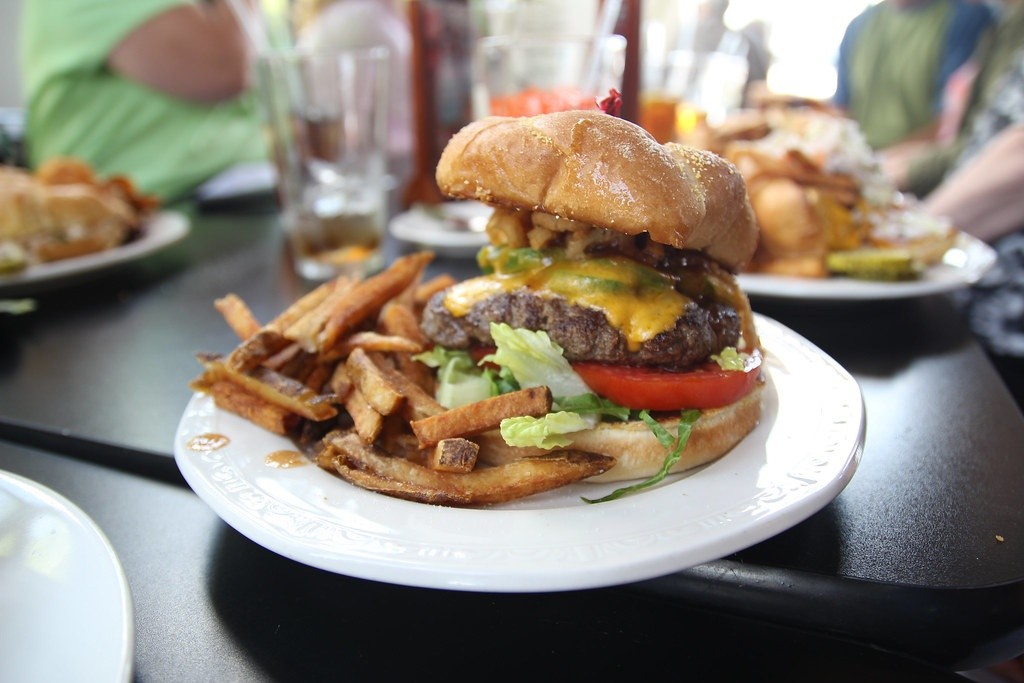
[418,110,763,504]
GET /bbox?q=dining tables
[0,190,1024,683]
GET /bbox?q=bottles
[297,3,417,194]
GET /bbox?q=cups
[253,48,392,278]
[470,34,626,122]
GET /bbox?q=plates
[197,163,278,201]
[733,215,999,300]
[0,209,192,293]
[174,310,867,593]
[0,470,135,683]
[389,201,498,258]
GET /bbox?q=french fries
[192,251,615,505]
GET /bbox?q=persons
[669,0,1024,414]
[19,0,301,216]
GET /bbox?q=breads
[0,160,135,272]
